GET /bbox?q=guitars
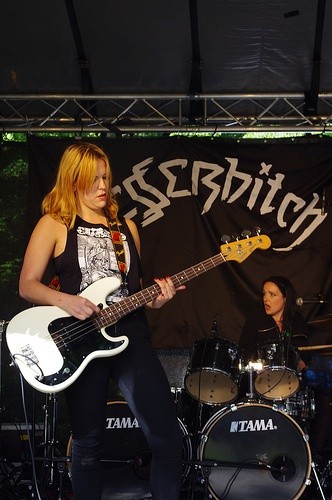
[4,225,274,393]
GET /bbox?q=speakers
[0,323,54,420]
[157,347,197,391]
[0,422,51,478]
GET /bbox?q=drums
[271,382,321,421]
[64,400,192,499]
[198,394,264,434]
[197,402,313,500]
[170,387,192,431]
[183,334,243,407]
[250,329,301,403]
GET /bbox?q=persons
[238,276,311,377]
[20,143,185,500]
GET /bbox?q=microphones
[296,298,326,305]
[136,457,150,468]
[211,320,217,334]
